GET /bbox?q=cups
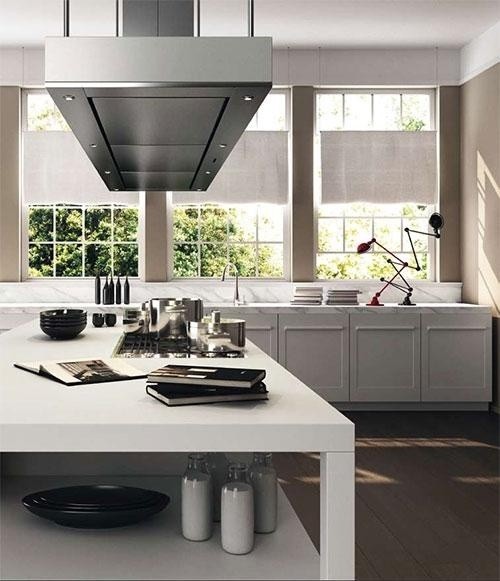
[92,313,117,328]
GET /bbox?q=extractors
[44,0,273,192]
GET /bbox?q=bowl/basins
[40,309,87,340]
[122,302,150,334]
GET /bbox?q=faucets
[221,264,238,299]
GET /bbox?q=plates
[21,484,170,529]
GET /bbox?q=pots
[184,312,244,354]
[150,297,202,332]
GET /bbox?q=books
[146,383,270,407]
[147,363,267,388]
[292,285,361,306]
[15,356,147,385]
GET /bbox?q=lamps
[356,211,444,306]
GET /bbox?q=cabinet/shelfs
[237,313,492,404]
[0,318,357,580]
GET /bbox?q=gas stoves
[113,330,245,359]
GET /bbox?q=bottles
[182,453,213,542]
[94,271,130,305]
[249,454,278,535]
[195,310,231,352]
[221,464,253,556]
